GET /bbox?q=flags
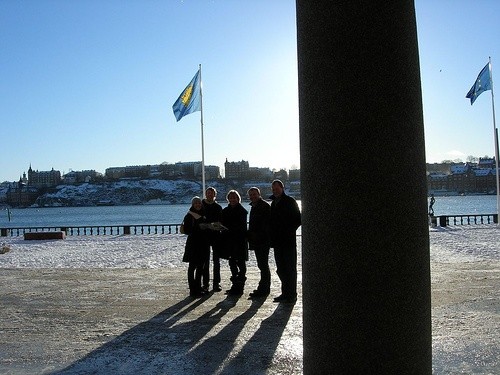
[172,68,201,121]
[465,62,493,105]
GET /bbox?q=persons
[217,190,248,297]
[428,193,436,214]
[270,179,302,302]
[248,186,270,298]
[182,187,223,298]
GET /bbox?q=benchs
[24,231,66,240]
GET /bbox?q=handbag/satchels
[180,224,186,234]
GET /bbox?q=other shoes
[249,290,268,297]
[274,293,297,303]
[226,286,243,295]
[190,285,210,297]
[213,285,222,291]
